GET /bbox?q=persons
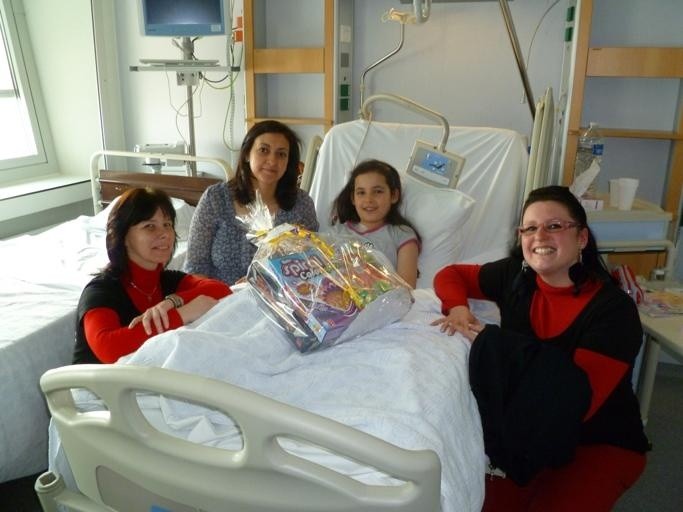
[179,119,319,287]
[71,187,232,362]
[320,159,420,290]
[429,185,653,510]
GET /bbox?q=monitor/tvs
[136,0,233,36]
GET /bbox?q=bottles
[572,120,605,193]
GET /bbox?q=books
[637,291,683,318]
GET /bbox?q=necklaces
[127,279,158,300]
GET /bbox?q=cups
[608,177,640,212]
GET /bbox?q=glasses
[521,220,579,234]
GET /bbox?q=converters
[236,30,243,41]
[237,17,243,27]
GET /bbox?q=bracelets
[164,294,184,308]
